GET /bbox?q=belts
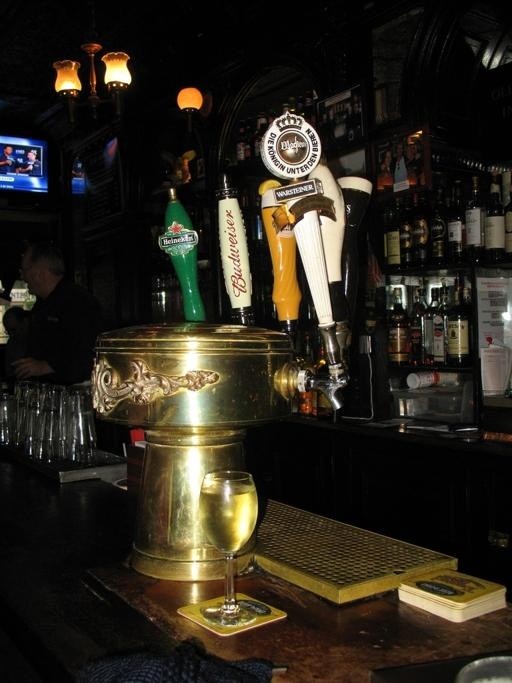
[194,471,260,628]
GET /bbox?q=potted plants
[375,125,432,194]
[314,82,365,161]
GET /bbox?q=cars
[1,381,99,467]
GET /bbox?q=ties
[0,134,59,207]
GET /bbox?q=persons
[375,132,427,189]
[14,147,42,178]
[0,144,20,174]
[10,245,110,391]
[1,304,30,383]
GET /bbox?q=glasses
[53,39,133,122]
[174,85,205,130]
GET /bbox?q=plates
[384,127,512,443]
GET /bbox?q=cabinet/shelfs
[389,282,471,367]
[238,92,319,162]
[376,168,510,273]
